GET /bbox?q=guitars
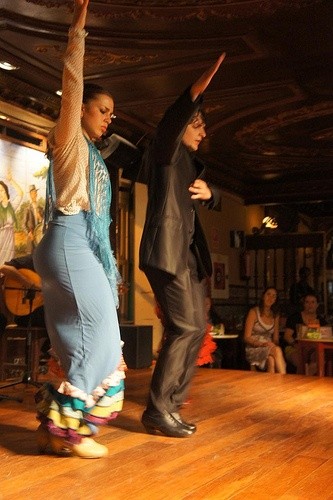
[3,269,127,316]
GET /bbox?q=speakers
[100,134,135,169]
[120,325,153,369]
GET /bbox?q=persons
[289,265,315,314]
[243,287,287,374]
[206,307,226,368]
[283,292,329,376]
[32,0,126,459]
[138,52,226,439]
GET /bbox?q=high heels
[34,425,108,459]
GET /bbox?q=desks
[294,336,333,377]
[211,334,239,368]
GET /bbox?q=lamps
[252,215,279,236]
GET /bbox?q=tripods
[0,285,46,390]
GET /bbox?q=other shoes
[40,364,48,374]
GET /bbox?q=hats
[26,184,39,193]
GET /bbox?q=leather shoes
[141,410,197,438]
